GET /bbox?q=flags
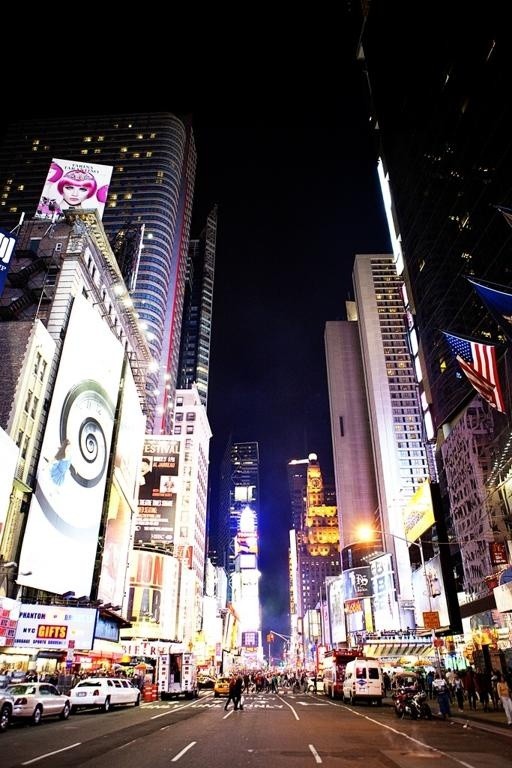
[345,600,362,614]
[459,274,512,325]
[437,325,507,416]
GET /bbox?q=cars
[199,644,386,706]
[0,667,157,732]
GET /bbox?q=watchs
[164,476,175,494]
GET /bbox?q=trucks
[152,649,201,700]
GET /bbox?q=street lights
[0,558,34,576]
[264,629,295,646]
[358,518,444,681]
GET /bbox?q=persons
[224,676,236,711]
[39,166,98,222]
[0,661,147,695]
[138,458,154,496]
[231,678,245,710]
[241,670,323,695]
[380,664,512,730]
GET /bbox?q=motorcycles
[393,687,434,721]
